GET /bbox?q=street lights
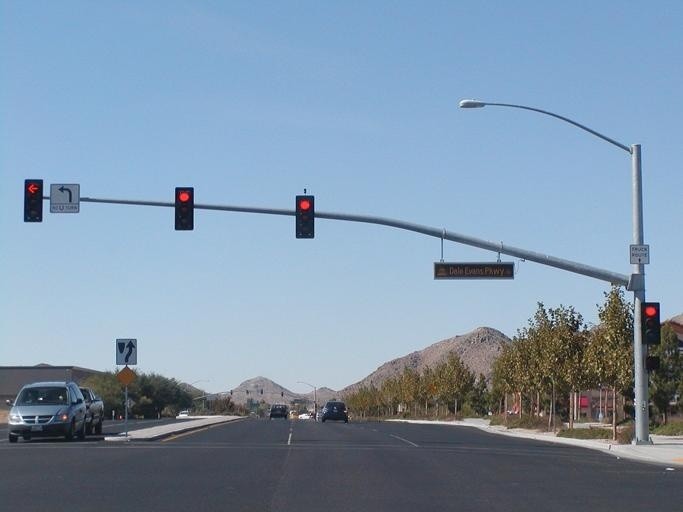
[459,100,649,445]
[296,381,316,421]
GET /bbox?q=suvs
[316,402,348,423]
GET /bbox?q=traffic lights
[175,187,194,230]
[296,196,314,238]
[24,179,42,222]
[640,302,661,345]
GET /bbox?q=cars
[298,413,313,420]
[179,411,189,416]
[6,382,104,443]
[269,405,289,419]
